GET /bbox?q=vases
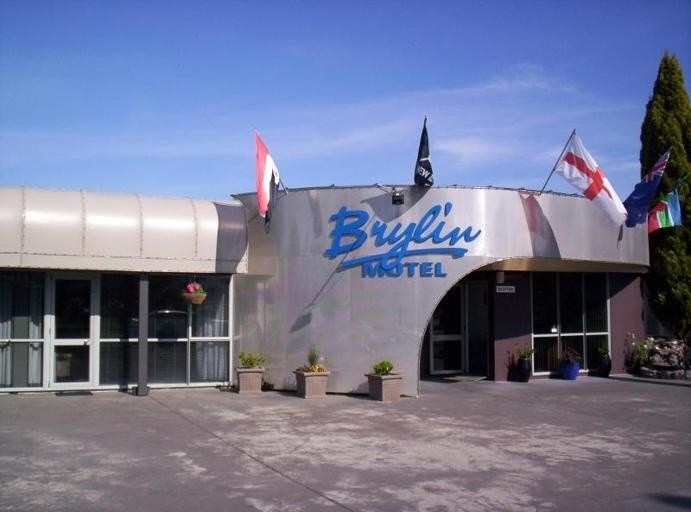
[560,360,579,380]
[180,294,207,304]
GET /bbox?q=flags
[557,134,628,227]
[254,131,278,235]
[620,150,671,227]
[647,188,682,234]
[412,121,434,188]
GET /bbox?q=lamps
[391,187,404,204]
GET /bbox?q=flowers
[181,282,205,294]
[561,352,576,362]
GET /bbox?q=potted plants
[597,347,611,377]
[234,353,266,393]
[293,345,330,398]
[517,348,536,382]
[366,361,402,401]
